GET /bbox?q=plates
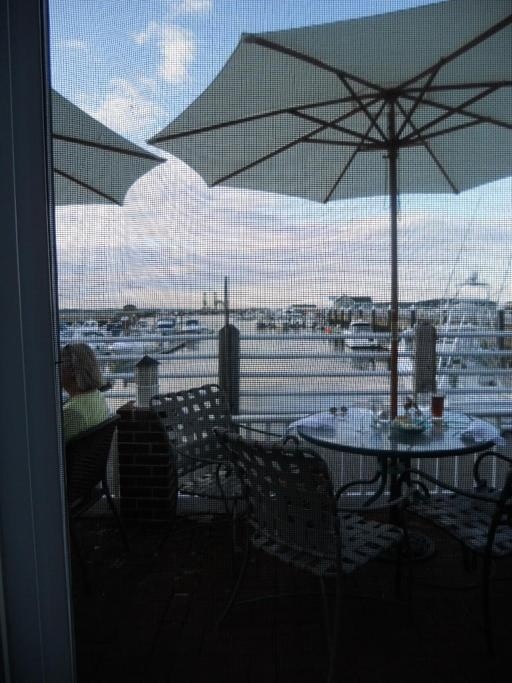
[391,416,425,433]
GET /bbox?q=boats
[59,312,204,352]
[389,269,512,426]
[345,344,376,373]
[343,319,378,348]
[231,308,321,332]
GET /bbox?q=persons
[57,343,116,512]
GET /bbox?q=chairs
[402,447,511,676]
[67,414,132,594]
[152,383,293,567]
[212,431,411,682]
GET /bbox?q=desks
[299,403,499,572]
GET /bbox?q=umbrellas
[46,84,168,211]
[142,0,511,421]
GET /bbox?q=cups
[431,392,447,419]
